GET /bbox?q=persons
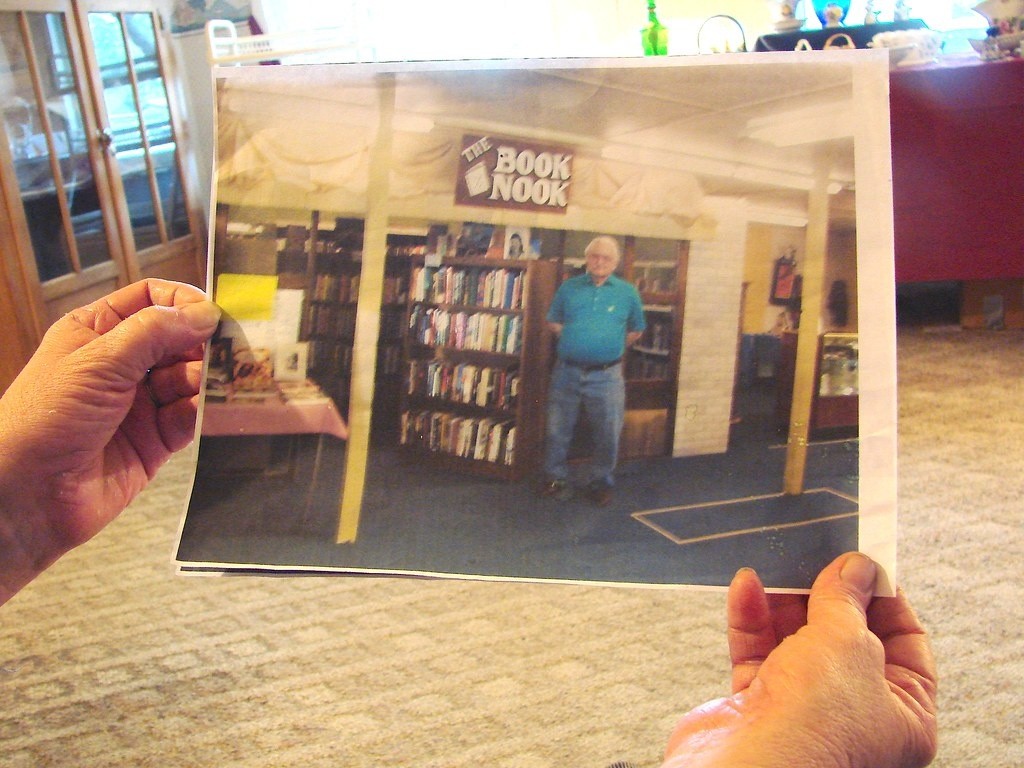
[0,276,938,768]
[538,236,648,506]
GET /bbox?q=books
[400,267,524,464]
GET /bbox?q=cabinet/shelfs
[202,208,691,482]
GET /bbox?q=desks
[202,376,348,528]
[890,51,1024,332]
